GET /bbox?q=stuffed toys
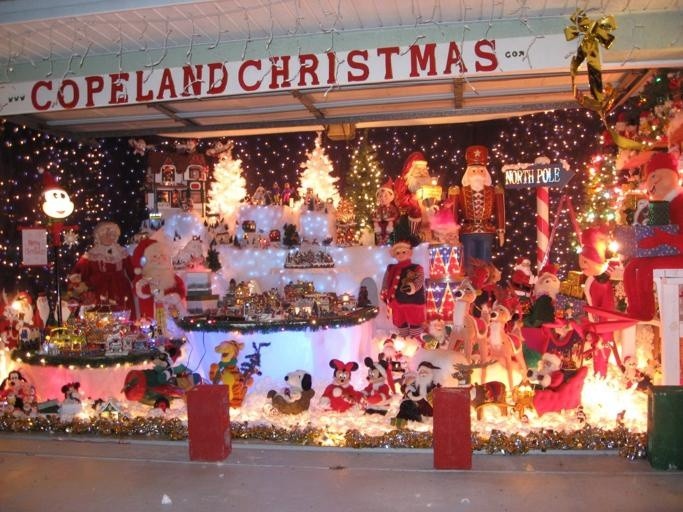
[1,70,681,424]
